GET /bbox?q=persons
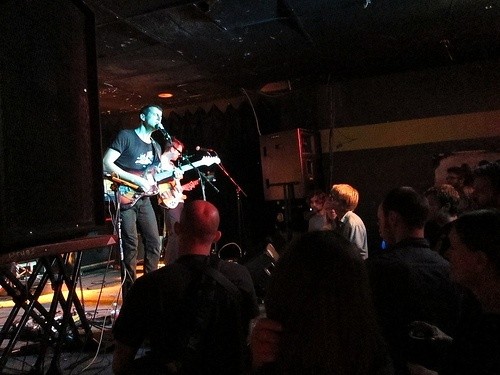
[369,185,459,324]
[158,137,182,266]
[101,106,185,299]
[112,200,256,375]
[308,191,328,231]
[407,209,500,375]
[247,231,397,375]
[321,183,368,261]
[448,160,499,209]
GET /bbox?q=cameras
[408,326,434,369]
[377,240,389,250]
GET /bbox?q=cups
[401,323,436,368]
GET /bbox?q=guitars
[116,155,221,209]
[157,180,199,209]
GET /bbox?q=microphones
[196,146,213,152]
[155,123,171,140]
[103,172,144,192]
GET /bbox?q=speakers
[0,0,105,255]
[260,128,317,200]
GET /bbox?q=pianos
[0,234,120,268]
